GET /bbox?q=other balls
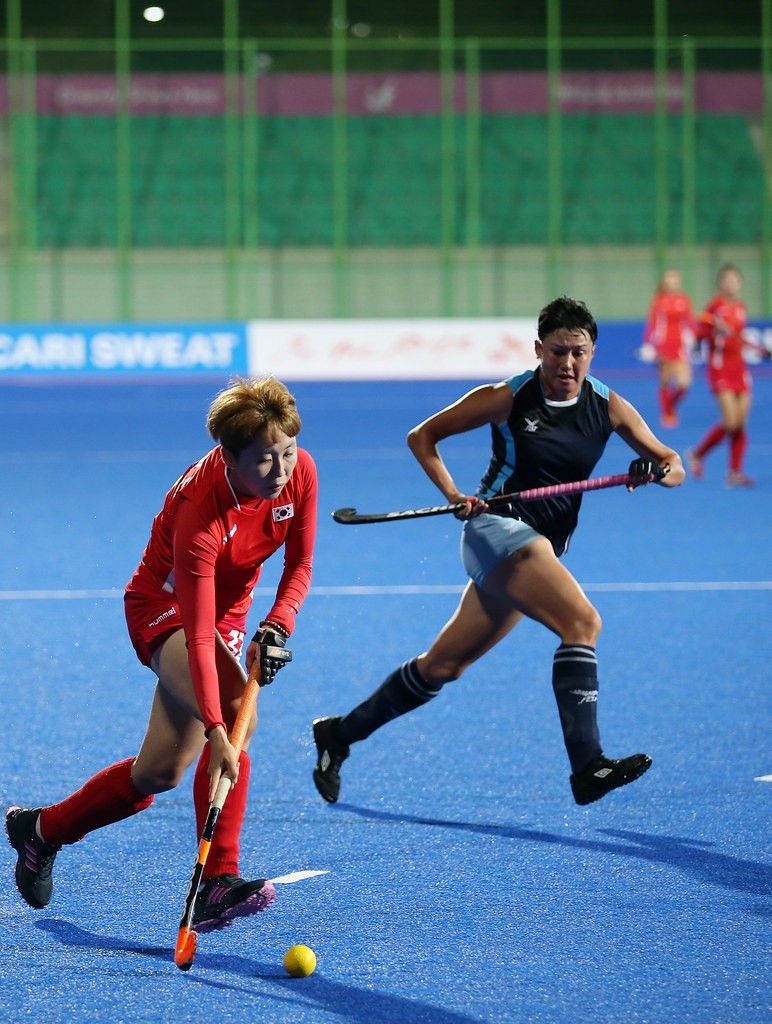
[284,945,316,977]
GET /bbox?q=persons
[636,269,704,427]
[683,258,772,489]
[309,297,679,808]
[5,377,317,932]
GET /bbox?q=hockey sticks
[171,657,268,971]
[325,472,651,528]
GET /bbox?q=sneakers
[312,716,350,804]
[188,873,276,933]
[3,806,62,910]
[569,753,652,805]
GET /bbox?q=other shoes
[724,471,752,486]
[683,445,703,476]
